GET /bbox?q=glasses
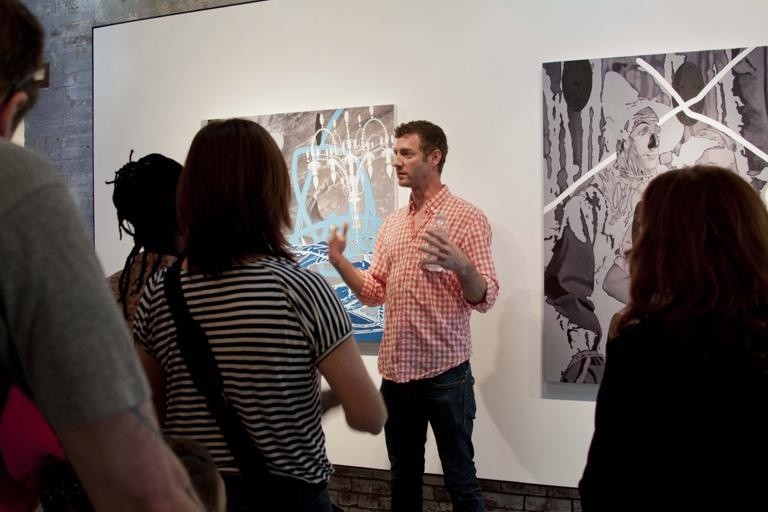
[5,62,53,100]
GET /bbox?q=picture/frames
[540,45,764,402]
[202,105,399,356]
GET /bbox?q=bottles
[425,215,449,271]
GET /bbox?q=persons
[0,0,207,512]
[104,148,184,425]
[131,117,389,512]
[327,119,500,511]
[578,164,768,511]
